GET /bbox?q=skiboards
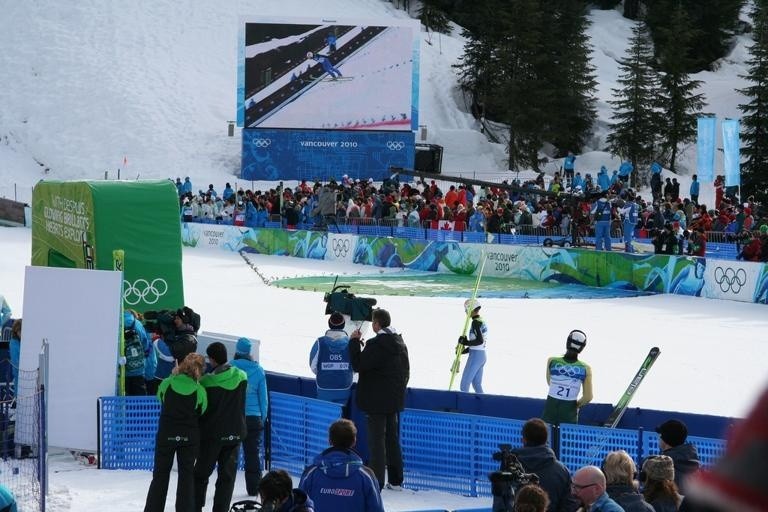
[582,346,660,463]
[447,252,489,391]
[112,249,125,461]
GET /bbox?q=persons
[306,50,342,80]
[299,417,385,511]
[257,469,314,512]
[124,304,269,512]
[455,298,487,394]
[491,417,700,511]
[348,309,410,493]
[8,318,22,399]
[326,31,336,53]
[541,329,593,425]
[310,312,354,405]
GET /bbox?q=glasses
[570,482,598,494]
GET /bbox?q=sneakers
[385,482,402,491]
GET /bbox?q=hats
[759,224,768,233]
[566,329,587,353]
[640,455,678,480]
[654,418,689,447]
[176,306,193,325]
[463,298,481,316]
[328,312,345,330]
[235,336,252,357]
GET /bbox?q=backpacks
[124,317,145,371]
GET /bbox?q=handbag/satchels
[217,428,242,448]
[162,420,199,447]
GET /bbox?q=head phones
[638,455,658,482]
